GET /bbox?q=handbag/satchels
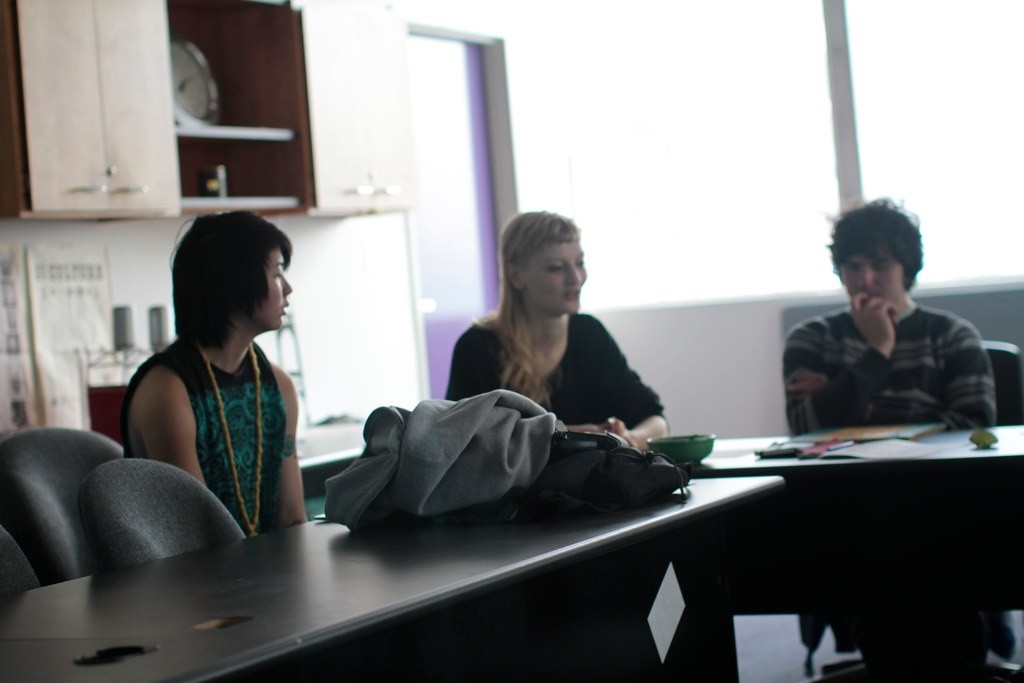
[500,432,693,527]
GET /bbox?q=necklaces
[201,341,262,538]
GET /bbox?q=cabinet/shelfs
[0,0,407,221]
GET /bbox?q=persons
[442,211,671,453]
[118,210,308,537]
[782,198,996,683]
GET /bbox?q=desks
[0,475,786,683]
[661,424,1024,683]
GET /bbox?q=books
[790,421,948,440]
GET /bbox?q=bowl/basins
[645,435,717,467]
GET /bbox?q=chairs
[0,429,127,587]
[798,340,1024,683]
[79,458,250,577]
